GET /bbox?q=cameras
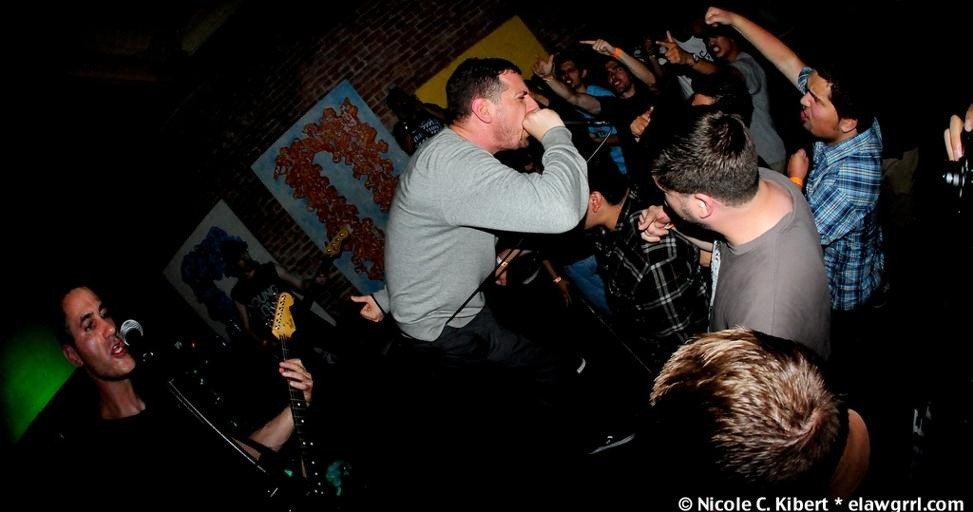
[400,95,416,113]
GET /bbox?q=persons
[41,269,316,512]
[213,237,341,378]
[345,4,923,512]
[941,102,972,163]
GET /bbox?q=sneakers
[582,427,636,455]
[533,354,587,383]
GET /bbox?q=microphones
[562,119,611,129]
[119,318,154,365]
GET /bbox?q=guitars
[264,292,353,511]
